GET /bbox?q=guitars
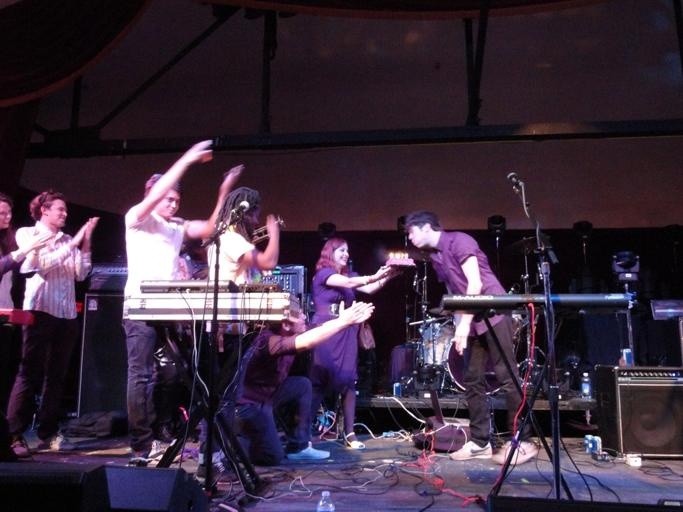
[415,364,467,451]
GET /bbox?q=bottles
[581,372,591,398]
[253,265,281,285]
[315,489,333,512]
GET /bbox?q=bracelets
[377,280,383,287]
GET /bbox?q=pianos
[442,294,635,309]
[140,282,279,292]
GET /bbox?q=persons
[404,211,539,464]
[198,188,280,463]
[122,141,242,463]
[1,190,98,464]
[234,301,375,463]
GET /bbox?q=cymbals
[431,308,455,316]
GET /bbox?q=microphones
[408,316,453,325]
[231,200,250,217]
[506,172,525,187]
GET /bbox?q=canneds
[620,347,633,369]
[393,382,402,398]
[584,434,593,455]
[383,431,395,438]
[590,435,601,453]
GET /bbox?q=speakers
[0,461,114,511]
[596,375,683,457]
[99,463,209,511]
[77,293,128,419]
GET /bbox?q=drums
[447,337,510,394]
[391,340,423,384]
[424,318,456,369]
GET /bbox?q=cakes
[385,251,417,268]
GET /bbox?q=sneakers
[449,440,493,460]
[37,433,78,453]
[285,439,331,461]
[492,440,539,466]
[196,463,241,485]
[10,435,30,459]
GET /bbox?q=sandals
[341,430,365,450]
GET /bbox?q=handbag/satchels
[358,320,376,351]
